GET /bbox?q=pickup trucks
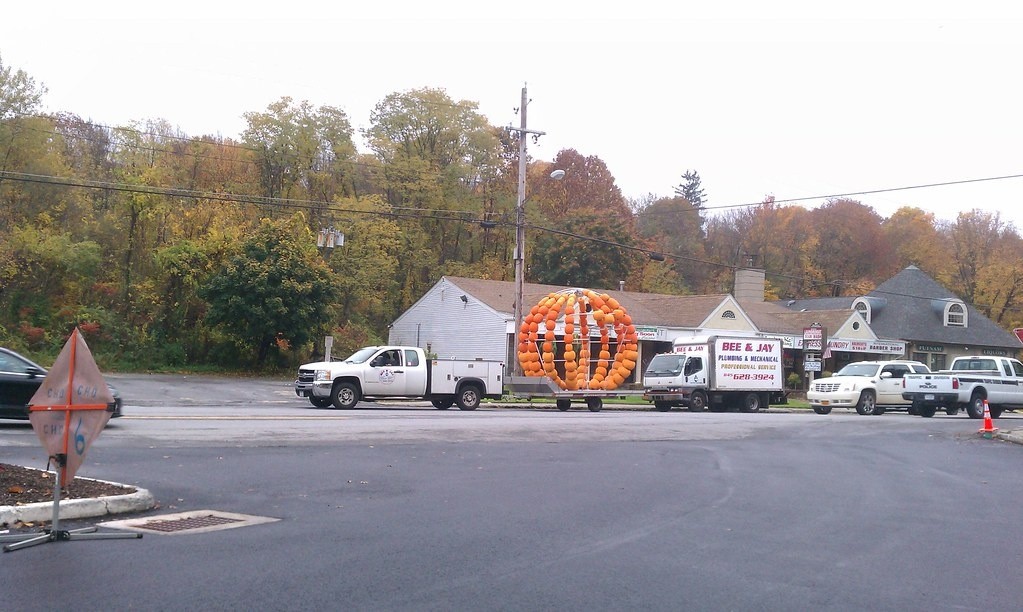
[902,356,1023,419]
[295,346,504,411]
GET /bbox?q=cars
[0,347,122,420]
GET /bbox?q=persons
[383,353,390,359]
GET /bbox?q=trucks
[643,335,789,413]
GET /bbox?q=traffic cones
[979,400,998,432]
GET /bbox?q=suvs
[806,360,932,415]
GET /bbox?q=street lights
[514,170,566,375]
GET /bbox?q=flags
[823,342,831,359]
